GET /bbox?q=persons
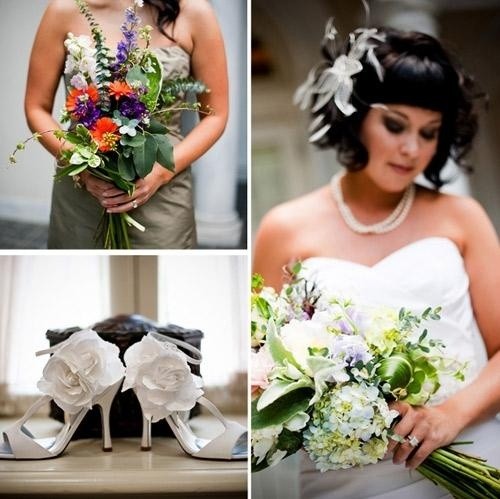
[252,28,500,499]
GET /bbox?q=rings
[131,201,138,209]
[407,436,419,448]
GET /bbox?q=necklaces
[330,165,415,236]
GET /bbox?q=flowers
[122,336,208,423]
[34,329,125,418]
[251,256,500,499]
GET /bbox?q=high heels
[0,328,126,460]
[121,330,248,461]
